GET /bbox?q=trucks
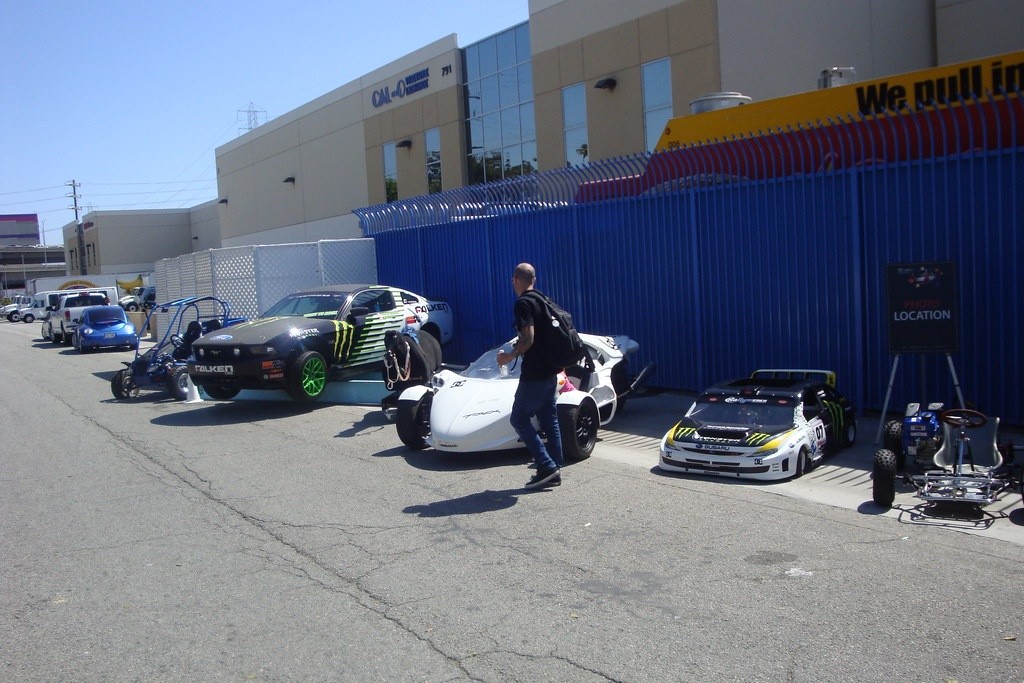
[0,294,36,323]
[119,286,155,312]
[19,286,118,324]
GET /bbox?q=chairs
[175,321,202,358]
[206,319,222,333]
[933,418,1003,470]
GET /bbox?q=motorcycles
[111,295,247,402]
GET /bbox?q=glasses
[511,278,514,284]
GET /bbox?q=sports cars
[394,330,639,460]
[659,369,858,483]
[72,305,139,353]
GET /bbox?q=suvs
[40,293,112,345]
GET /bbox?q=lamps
[283,177,294,184]
[219,198,227,203]
[395,140,411,147]
[593,79,616,88]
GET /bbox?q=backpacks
[524,290,586,376]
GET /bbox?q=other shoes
[524,464,559,487]
[530,474,561,486]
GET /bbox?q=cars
[34,244,49,249]
[186,283,454,402]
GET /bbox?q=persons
[498,263,564,488]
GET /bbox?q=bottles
[498,349,508,378]
